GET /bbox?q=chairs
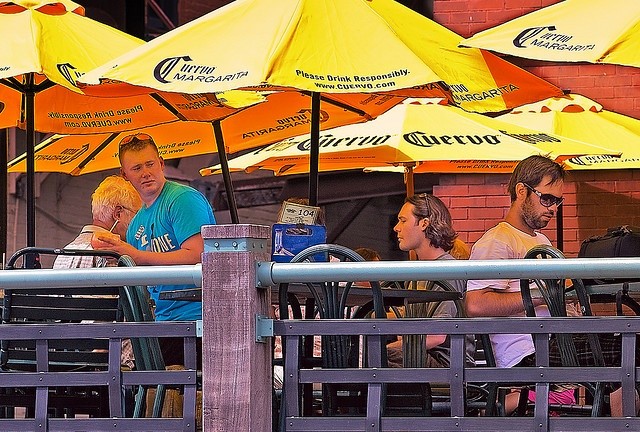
[475,317,532,417]
[522,245,608,416]
[111,252,202,417]
[0,245,126,419]
[276,241,435,414]
[379,279,502,418]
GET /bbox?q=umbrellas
[362,89,640,254]
[200,101,623,261]
[0,1,147,269]
[74,1,574,205]
[458,0,639,70]
[7,95,405,225]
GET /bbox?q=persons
[372,193,477,401]
[93,133,219,372]
[52,174,157,368]
[273,196,365,391]
[465,155,640,418]
[345,247,382,286]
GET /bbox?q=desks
[155,281,462,414]
[527,280,639,329]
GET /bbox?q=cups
[90,230,121,249]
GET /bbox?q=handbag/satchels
[577,225,640,284]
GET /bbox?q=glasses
[119,134,158,159]
[419,193,430,217]
[523,182,565,207]
